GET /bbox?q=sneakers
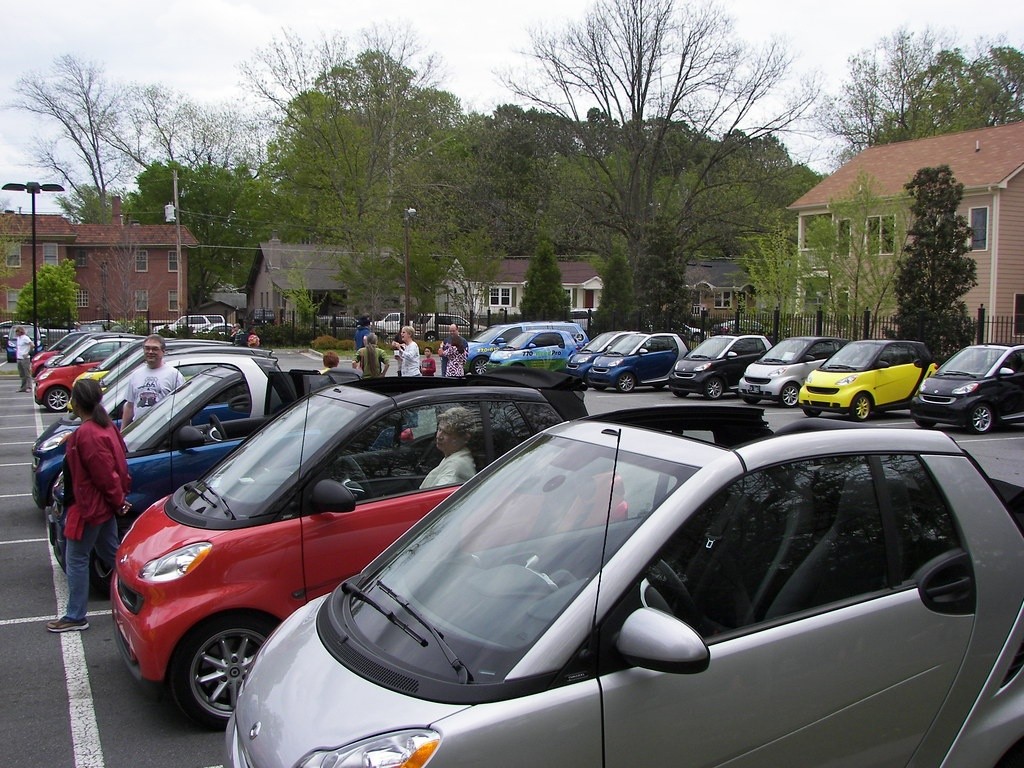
[46,617,89,632]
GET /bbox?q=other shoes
[26,388,31,393]
[16,389,23,392]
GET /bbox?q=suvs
[467,320,590,376]
[413,312,488,342]
[245,309,276,330]
[153,314,226,334]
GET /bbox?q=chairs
[674,460,815,642]
[767,464,917,621]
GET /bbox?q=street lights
[2,182,65,355]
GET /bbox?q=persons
[46,378,134,632]
[14,326,34,393]
[318,317,469,377]
[231,323,257,348]
[418,407,478,492]
[118,334,187,433]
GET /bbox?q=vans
[569,310,593,330]
[310,315,359,334]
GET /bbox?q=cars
[192,323,233,336]
[739,337,816,420]
[909,343,1024,434]
[646,319,707,343]
[711,320,771,336]
[798,340,939,422]
[73,320,126,332]
[225,400,1024,767]
[369,312,413,334]
[109,374,588,731]
[0,320,47,362]
[30,320,148,412]
[565,330,691,393]
[487,329,580,372]
[670,335,773,400]
[32,339,362,600]
